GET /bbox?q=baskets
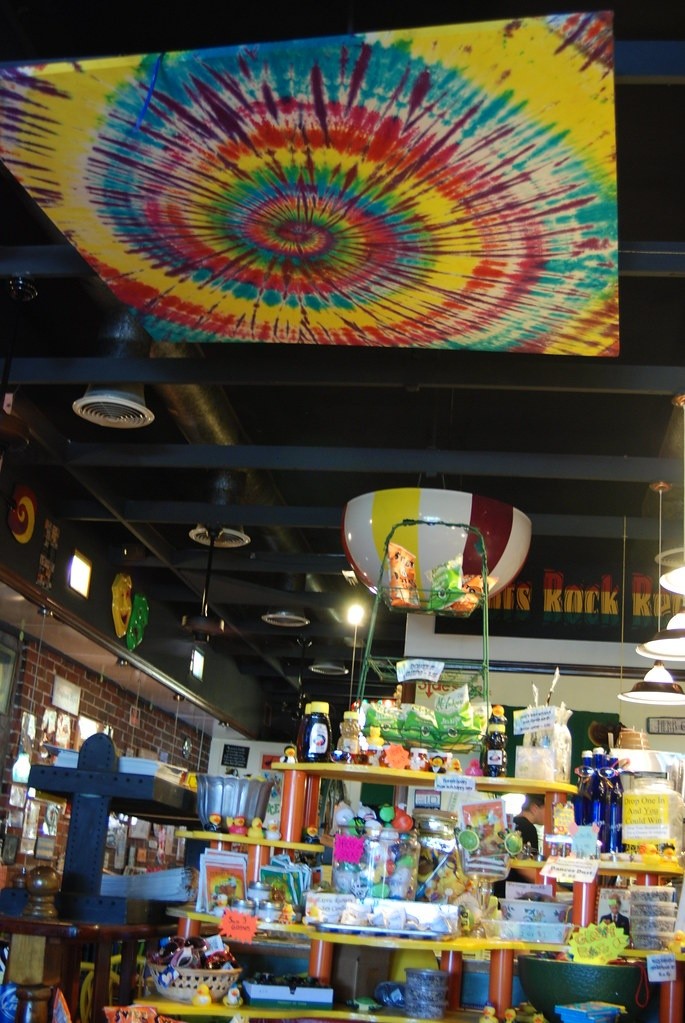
[146,957,242,1004]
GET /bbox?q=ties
[613,914,617,922]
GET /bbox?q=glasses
[574,766,620,778]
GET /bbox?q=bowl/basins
[404,968,450,1020]
[498,899,568,925]
[631,886,678,950]
[516,957,658,1023]
[196,774,276,831]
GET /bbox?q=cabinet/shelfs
[136,761,685,1023]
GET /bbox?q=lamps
[617,395,685,707]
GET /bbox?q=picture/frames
[593,885,631,948]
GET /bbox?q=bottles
[571,747,624,853]
[296,701,332,763]
[481,706,508,777]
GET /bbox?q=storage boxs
[506,882,552,902]
[555,1001,626,1023]
[484,918,573,945]
[243,980,334,1009]
[497,894,572,923]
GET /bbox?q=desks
[0,886,219,1023]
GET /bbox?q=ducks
[228,816,247,835]
[280,746,297,764]
[209,814,221,832]
[247,817,264,839]
[266,818,282,842]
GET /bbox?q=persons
[600,894,629,935]
[493,793,547,907]
